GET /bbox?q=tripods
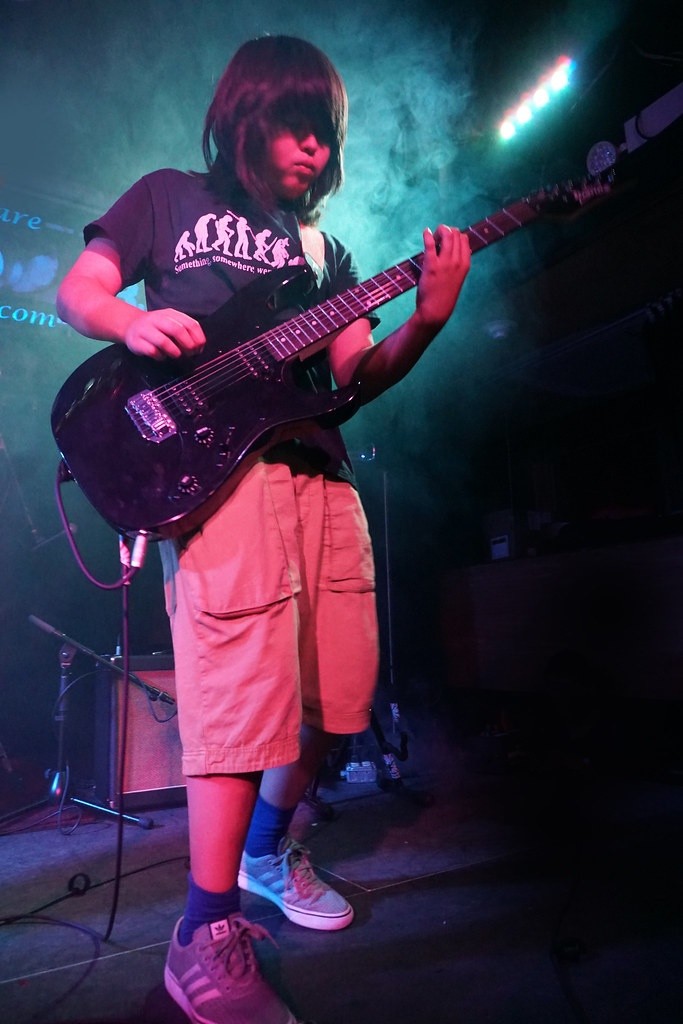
[0,614,175,828]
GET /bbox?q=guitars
[50,166,620,544]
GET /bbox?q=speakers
[73,652,187,811]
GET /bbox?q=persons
[56,33,469,1024]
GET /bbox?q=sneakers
[165,905,298,1024]
[237,834,353,930]
